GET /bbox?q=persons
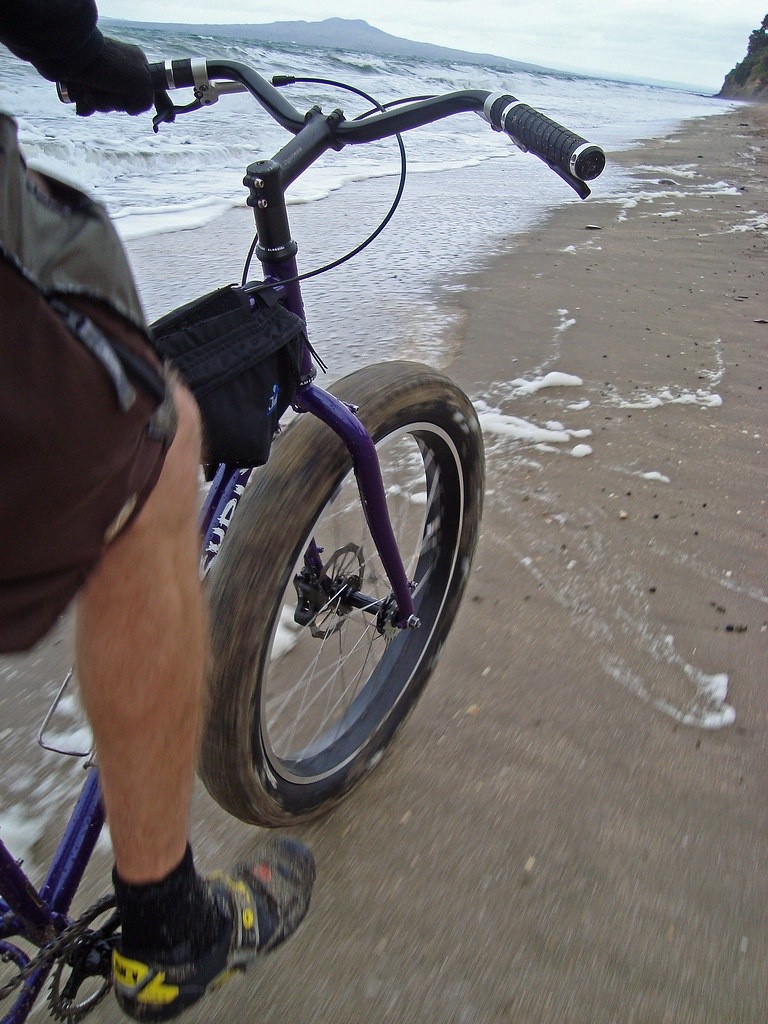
[0,0,315,1021]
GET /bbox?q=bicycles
[0,57,605,1024]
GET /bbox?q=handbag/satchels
[147,277,328,482]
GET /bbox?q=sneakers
[112,833,316,1024]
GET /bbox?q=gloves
[70,36,153,116]
